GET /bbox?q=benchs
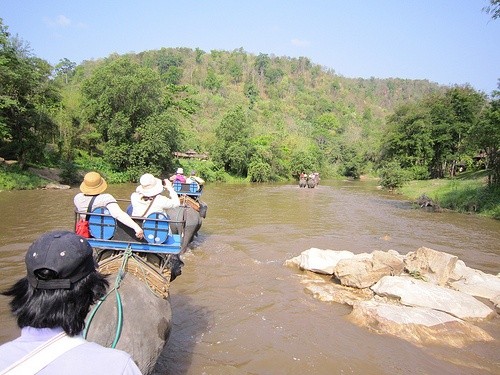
[172,177,204,195]
[74,198,186,254]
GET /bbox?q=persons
[0,229,144,375]
[186,170,205,191]
[74,171,144,240]
[170,168,187,183]
[300,171,320,181]
[131,172,180,238]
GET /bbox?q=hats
[80,172,107,195]
[136,174,163,197]
[25,230,99,289]
[177,168,183,174]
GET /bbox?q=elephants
[298,178,307,189]
[79,248,174,375]
[307,179,315,188]
[314,176,320,185]
[165,197,208,257]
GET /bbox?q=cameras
[162,180,166,186]
